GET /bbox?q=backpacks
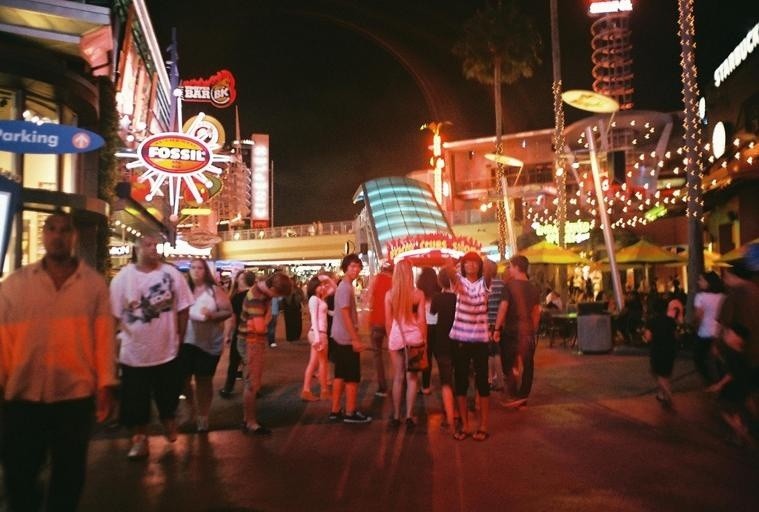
[404,342,430,372]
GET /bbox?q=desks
[552,312,578,347]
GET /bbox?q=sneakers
[300,391,320,402]
[177,414,193,434]
[406,417,414,433]
[328,408,344,421]
[127,434,150,461]
[389,414,400,428]
[166,420,177,442]
[375,387,388,397]
[196,415,209,432]
[320,391,332,401]
[343,411,373,425]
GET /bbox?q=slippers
[454,430,474,440]
[472,430,490,441]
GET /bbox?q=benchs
[571,303,608,349]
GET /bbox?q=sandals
[241,423,273,436]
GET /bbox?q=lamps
[699,96,709,126]
[712,120,734,161]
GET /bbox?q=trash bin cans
[577,301,614,354]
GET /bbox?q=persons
[693,272,726,384]
[446,253,495,441]
[237,273,292,431]
[534,270,607,311]
[109,233,196,461]
[385,261,427,432]
[705,259,759,442]
[300,274,341,401]
[267,269,305,347]
[474,258,541,411]
[213,269,264,398]
[616,277,687,346]
[369,262,394,396]
[643,300,678,407]
[181,256,234,431]
[0,211,118,512]
[329,254,372,423]
[416,267,457,434]
